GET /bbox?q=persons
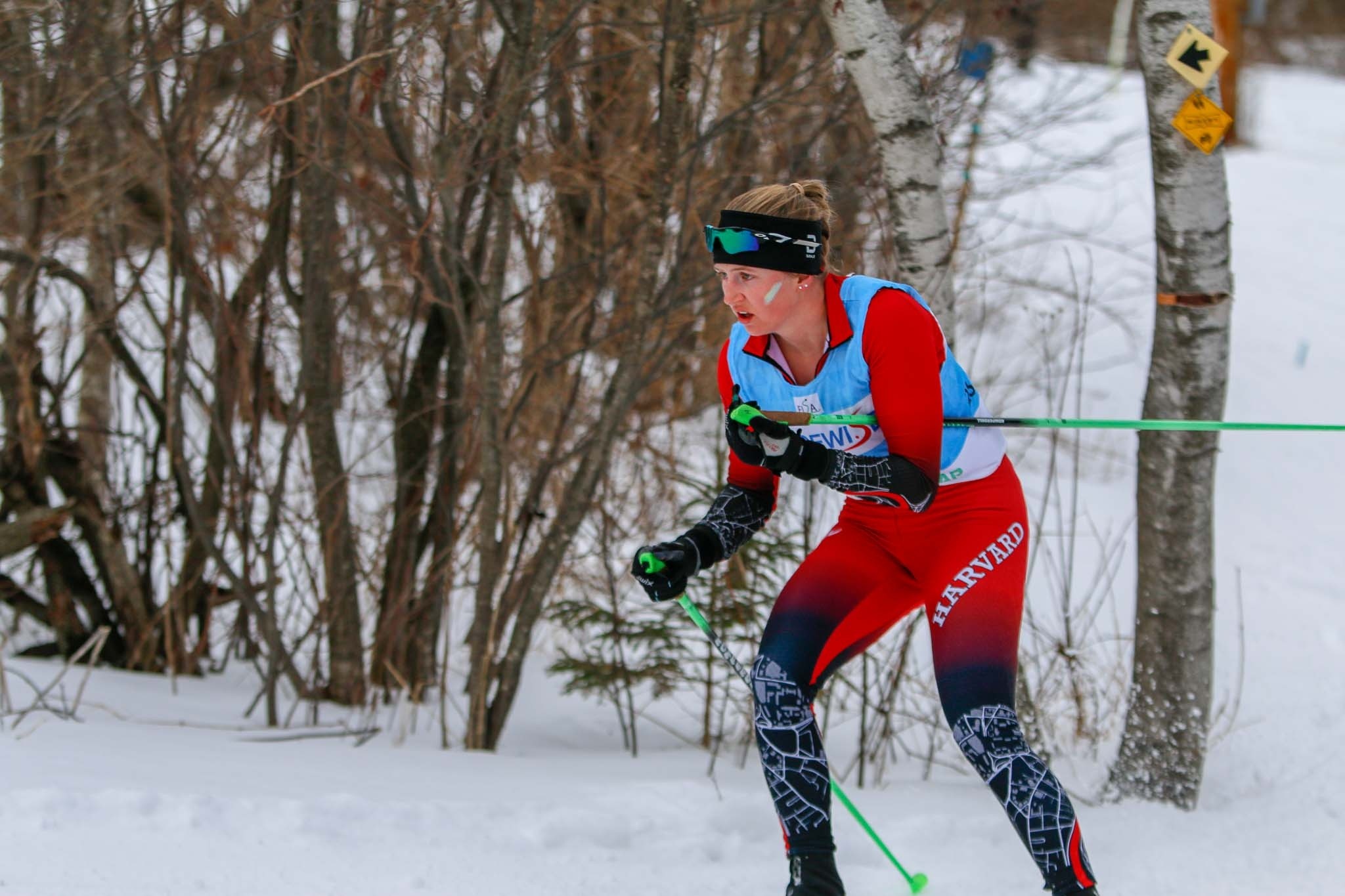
[629,178,1098,895]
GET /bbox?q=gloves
[726,401,835,484]
[631,534,701,602]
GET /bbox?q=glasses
[703,225,821,255]
